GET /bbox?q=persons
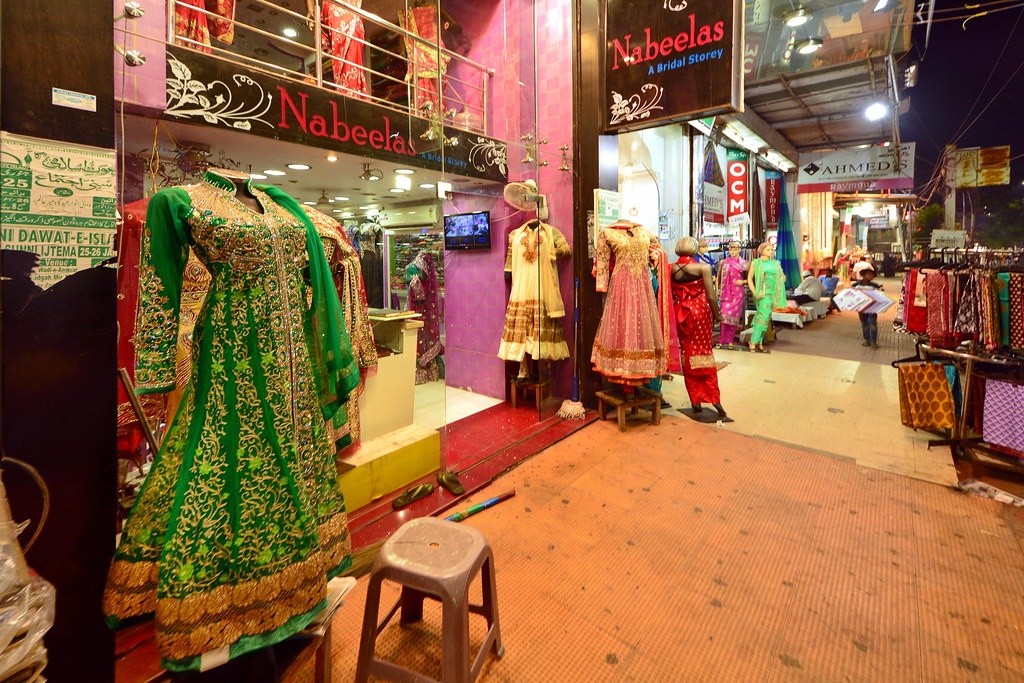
[589,220,668,400]
[668,237,735,422]
[305,0,370,102]
[883,252,897,278]
[715,242,749,349]
[851,270,884,348]
[818,269,839,296]
[801,234,810,270]
[105,167,356,683]
[496,218,571,387]
[748,242,787,353]
[694,237,718,297]
[397,0,462,121]
[852,257,875,283]
[341,220,359,253]
[789,270,825,306]
[359,219,383,309]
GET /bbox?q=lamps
[316,190,333,215]
[177,141,210,173]
[359,163,383,181]
[795,38,822,54]
[782,9,814,27]
[718,117,797,173]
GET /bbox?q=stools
[352,517,505,683]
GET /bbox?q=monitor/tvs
[443,210,491,250]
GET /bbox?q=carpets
[675,361,727,376]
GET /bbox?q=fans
[503,179,549,220]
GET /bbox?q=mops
[554,276,587,421]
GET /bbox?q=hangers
[891,342,952,368]
[891,247,1024,278]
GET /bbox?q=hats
[803,271,811,277]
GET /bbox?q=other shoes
[873,344,879,350]
[862,342,870,346]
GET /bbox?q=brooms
[336,488,516,579]
